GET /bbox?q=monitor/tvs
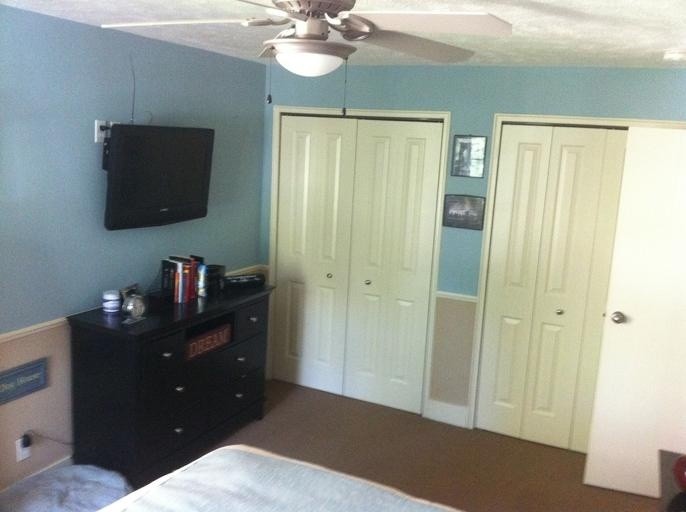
[102,124,216,230]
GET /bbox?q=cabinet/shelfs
[65,283,277,489]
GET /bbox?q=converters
[21,433,33,447]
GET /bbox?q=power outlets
[15,439,33,462]
[94,120,106,143]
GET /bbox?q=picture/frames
[451,135,487,178]
[443,194,485,230]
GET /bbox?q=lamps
[261,1,356,79]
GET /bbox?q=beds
[96,443,467,512]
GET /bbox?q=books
[160,255,206,303]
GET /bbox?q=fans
[101,0,475,68]
